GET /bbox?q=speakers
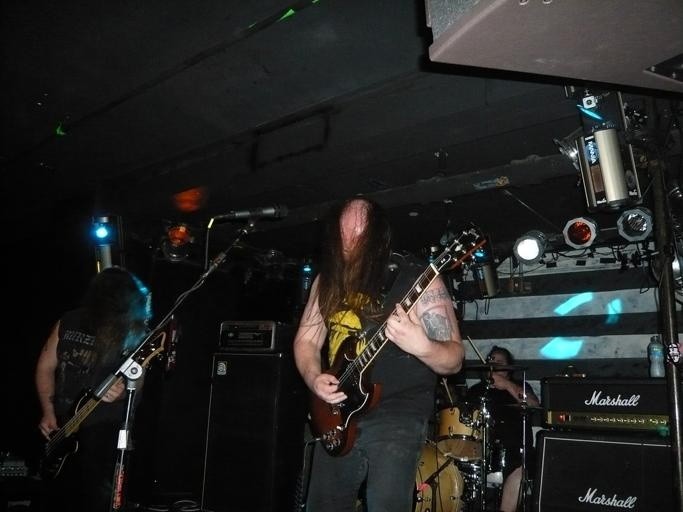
[531,430,683,512]
[472,258,501,299]
[573,128,630,214]
[198,351,304,511]
[85,214,129,274]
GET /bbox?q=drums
[436,402,495,460]
[414,438,485,512]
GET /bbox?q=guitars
[38,317,179,481]
[310,223,485,457]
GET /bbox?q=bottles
[649,335,666,378]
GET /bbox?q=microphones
[215,203,289,222]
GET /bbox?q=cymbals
[464,364,525,372]
[498,404,543,411]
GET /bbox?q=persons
[35,267,151,512]
[462,346,540,512]
[293,196,464,512]
